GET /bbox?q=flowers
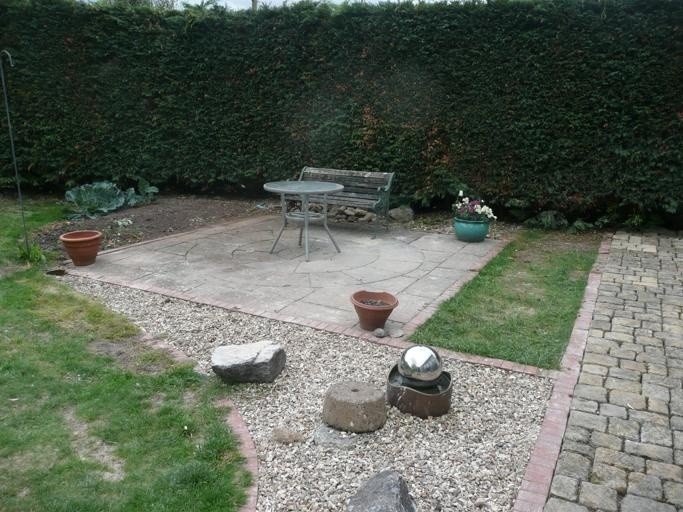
[452,189,498,222]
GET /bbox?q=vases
[454,217,489,242]
[351,290,399,331]
[59,230,103,266]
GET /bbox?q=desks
[263,181,344,261]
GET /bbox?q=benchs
[281,165,395,240]
